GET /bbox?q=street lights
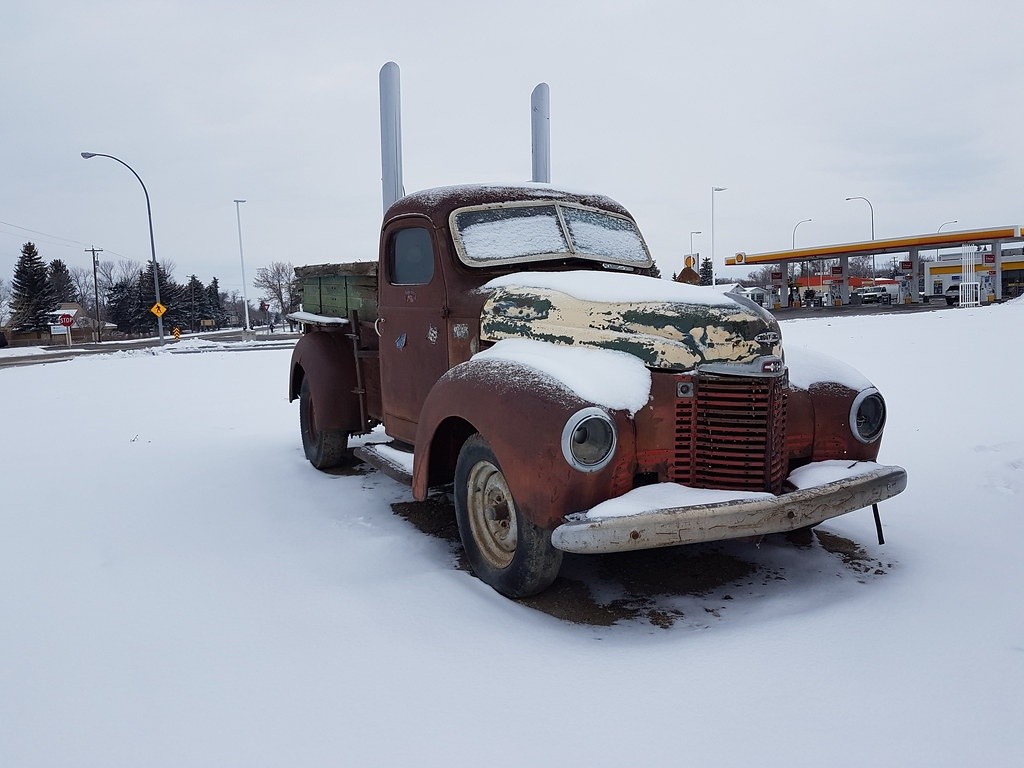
[690,231,701,269]
[233,198,253,331]
[791,219,813,286]
[709,186,728,290]
[845,196,875,287]
[80,151,167,347]
[935,220,958,261]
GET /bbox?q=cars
[944,284,978,306]
[848,286,888,303]
[243,321,253,330]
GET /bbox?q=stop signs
[59,313,74,326]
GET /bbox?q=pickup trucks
[281,181,909,601]
